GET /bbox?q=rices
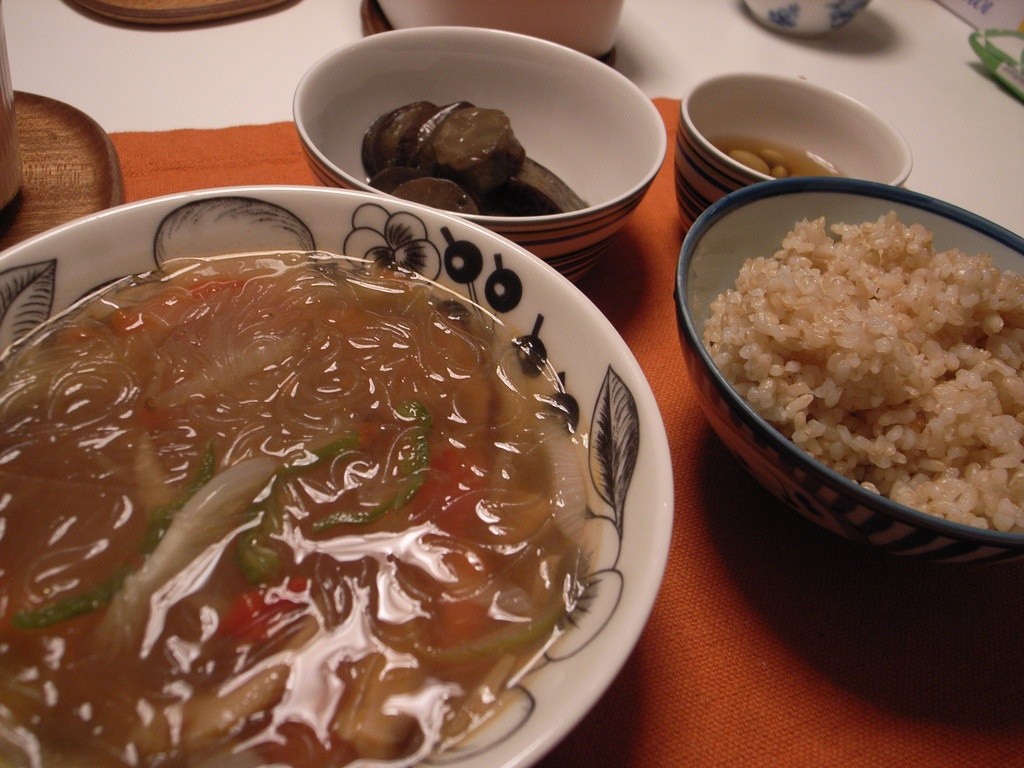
[702,208,1024,535]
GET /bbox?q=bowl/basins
[676,176,1024,571]
[0,183,674,768]
[291,25,669,289]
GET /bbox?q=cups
[743,0,873,38]
[673,73,912,253]
[368,0,623,58]
[0,2,24,215]
